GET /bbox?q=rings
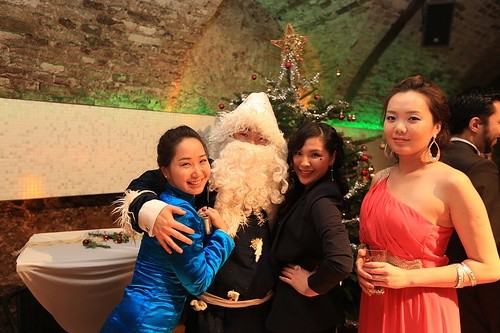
[298,265,300,271]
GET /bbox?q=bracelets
[462,262,477,287]
[454,263,466,289]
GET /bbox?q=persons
[273,122,354,333]
[99,125,235,333]
[431,84,500,333]
[355,75,500,333]
[112,90,283,333]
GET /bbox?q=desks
[16,228,143,333]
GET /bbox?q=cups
[364,249,387,294]
